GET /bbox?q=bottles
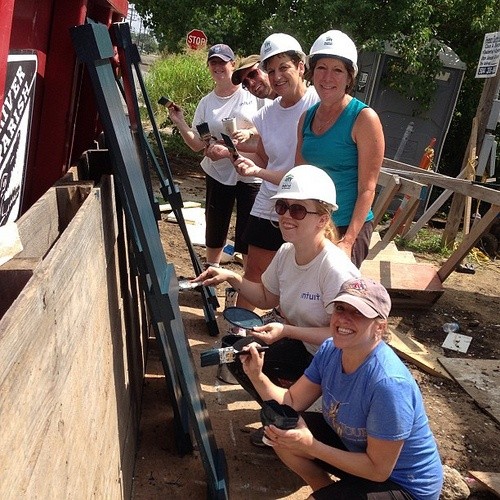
[443,322,460,332]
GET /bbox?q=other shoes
[249,427,267,446]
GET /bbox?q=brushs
[196,122,212,148]
[157,96,181,113]
[200,346,270,367]
[178,278,205,292]
[220,132,239,161]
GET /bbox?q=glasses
[242,69,259,89]
[275,199,318,220]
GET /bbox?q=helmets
[305,30,358,79]
[257,33,306,73]
[269,165,338,212]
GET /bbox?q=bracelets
[248,129,253,139]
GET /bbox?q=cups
[225,288,239,309]
[222,117,237,141]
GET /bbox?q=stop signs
[186,29,207,51]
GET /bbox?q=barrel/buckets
[217,334,246,385]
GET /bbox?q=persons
[168,43,257,288]
[189,165,364,446]
[295,30,385,272]
[202,33,320,337]
[262,278,443,500]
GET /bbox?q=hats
[208,44,234,62]
[231,54,261,85]
[324,276,391,320]
